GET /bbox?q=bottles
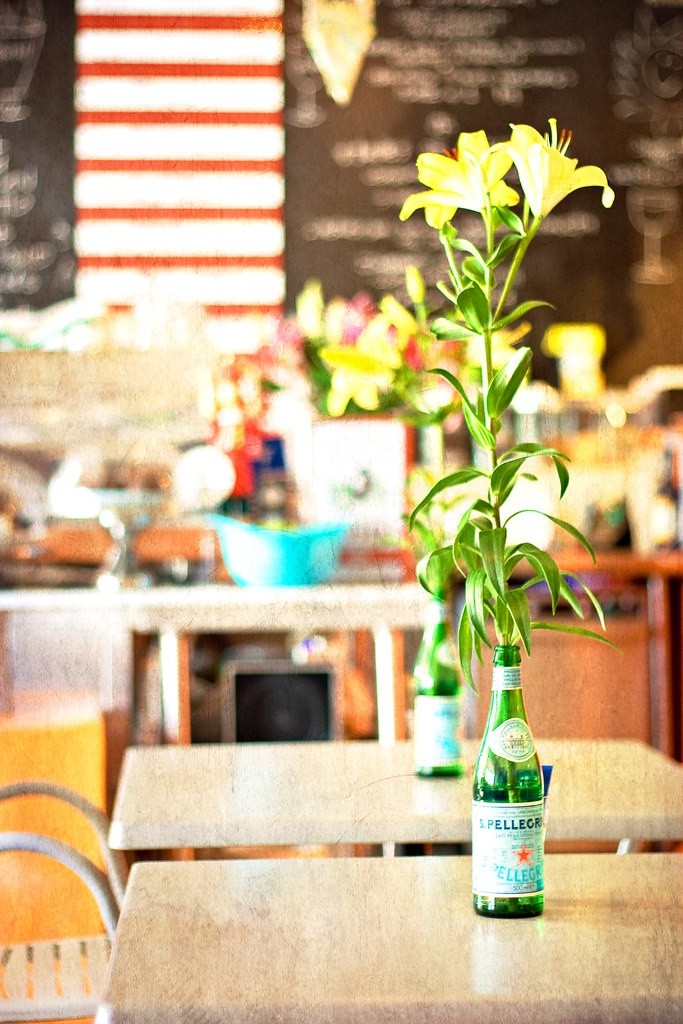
[412,603,462,778]
[472,645,545,918]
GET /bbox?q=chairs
[0,780,125,1024]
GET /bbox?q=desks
[107,744,683,857]
[79,853,683,1024]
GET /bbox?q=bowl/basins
[208,511,349,588]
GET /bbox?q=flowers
[292,264,474,578]
[396,117,623,698]
